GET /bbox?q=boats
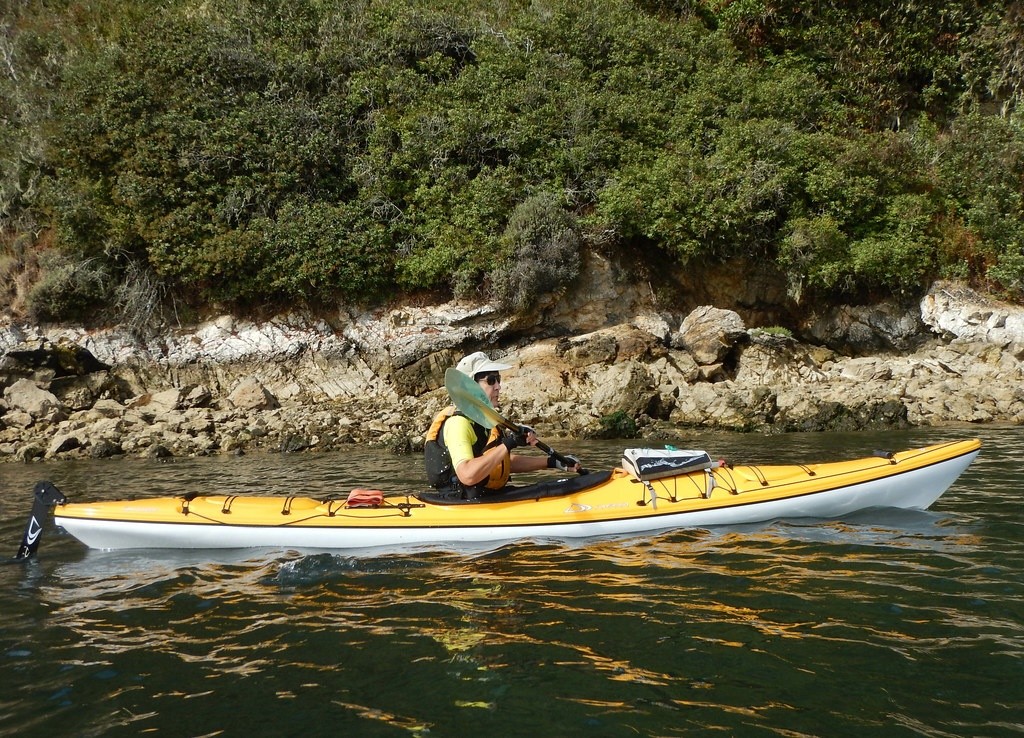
[18,438,984,555]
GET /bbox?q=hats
[455,352,514,381]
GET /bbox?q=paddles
[444,367,587,473]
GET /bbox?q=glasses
[480,375,501,385]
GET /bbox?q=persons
[424,352,582,501]
